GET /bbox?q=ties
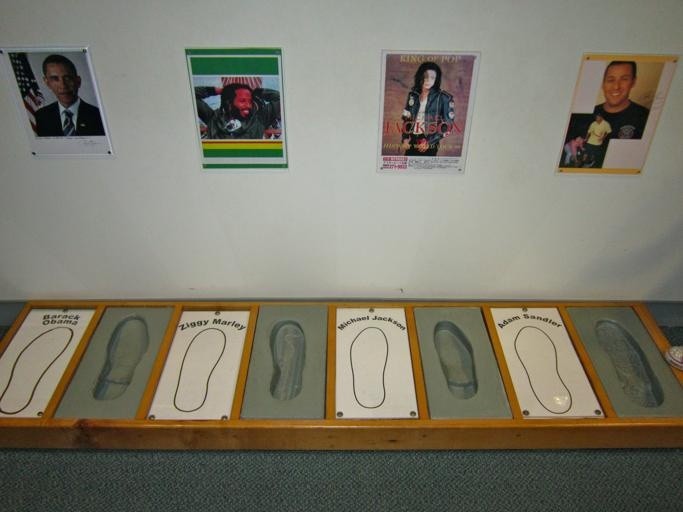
[63,111,76,136]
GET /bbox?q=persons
[593,59,649,139]
[573,152,596,169]
[579,111,613,167]
[400,59,456,157]
[193,82,281,140]
[562,133,585,168]
[35,54,105,138]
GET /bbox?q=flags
[8,51,47,137]
